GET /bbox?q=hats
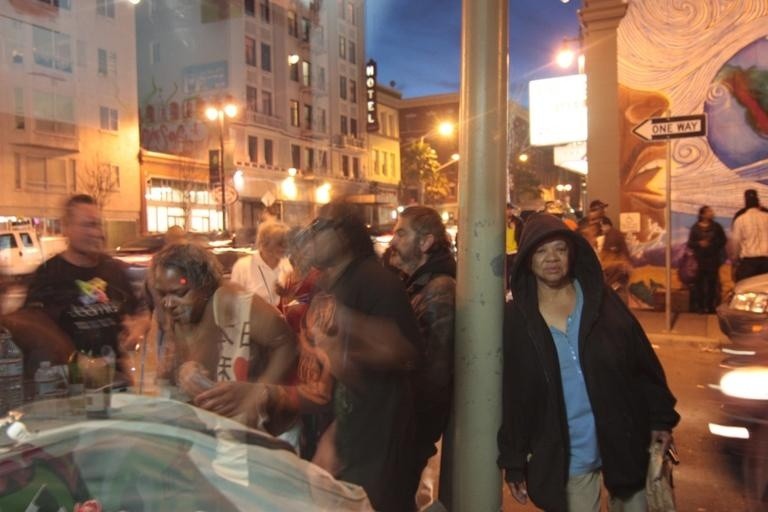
[587,199,610,211]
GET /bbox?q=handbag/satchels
[644,434,681,512]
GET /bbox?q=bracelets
[66,349,80,366]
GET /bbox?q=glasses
[310,216,344,232]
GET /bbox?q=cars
[112,230,257,284]
[713,269,768,461]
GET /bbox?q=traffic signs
[631,114,707,142]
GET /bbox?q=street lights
[417,119,458,208]
[204,103,239,229]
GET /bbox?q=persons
[493,211,680,511]
[145,220,186,369]
[73,474,145,511]
[230,216,295,313]
[144,239,297,428]
[385,204,457,510]
[292,200,430,512]
[731,186,768,284]
[277,225,324,329]
[15,191,151,405]
[505,199,633,308]
[1,248,40,417]
[686,205,727,304]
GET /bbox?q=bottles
[1,328,113,419]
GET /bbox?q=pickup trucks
[0,226,69,279]
